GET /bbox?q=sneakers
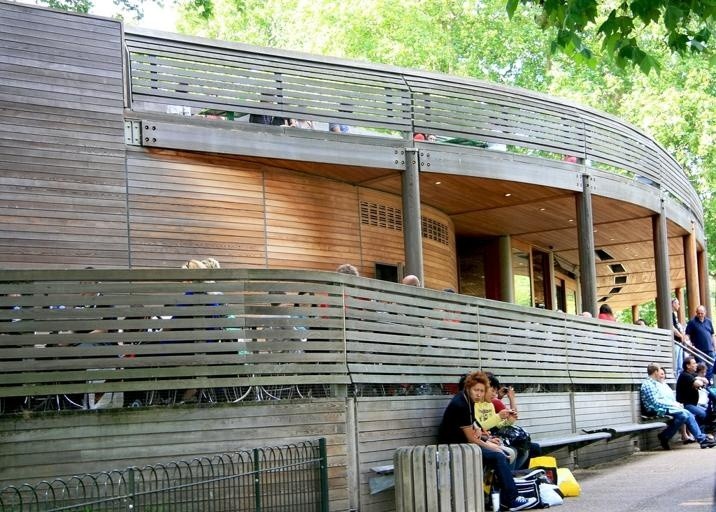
[700,435,716,449]
[657,433,673,450]
[508,495,538,512]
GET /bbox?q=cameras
[503,387,508,393]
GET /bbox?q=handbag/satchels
[495,425,531,447]
[510,456,582,508]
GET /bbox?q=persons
[641,358,716,450]
[686,304,716,386]
[414,131,436,142]
[438,370,537,511]
[634,173,654,184]
[1,257,647,410]
[563,154,577,163]
[250,89,354,134]
[546,470,553,482]
[671,297,686,371]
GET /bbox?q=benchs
[367,451,448,496]
[641,411,678,424]
[530,427,614,458]
[586,418,670,445]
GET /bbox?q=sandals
[683,437,696,444]
